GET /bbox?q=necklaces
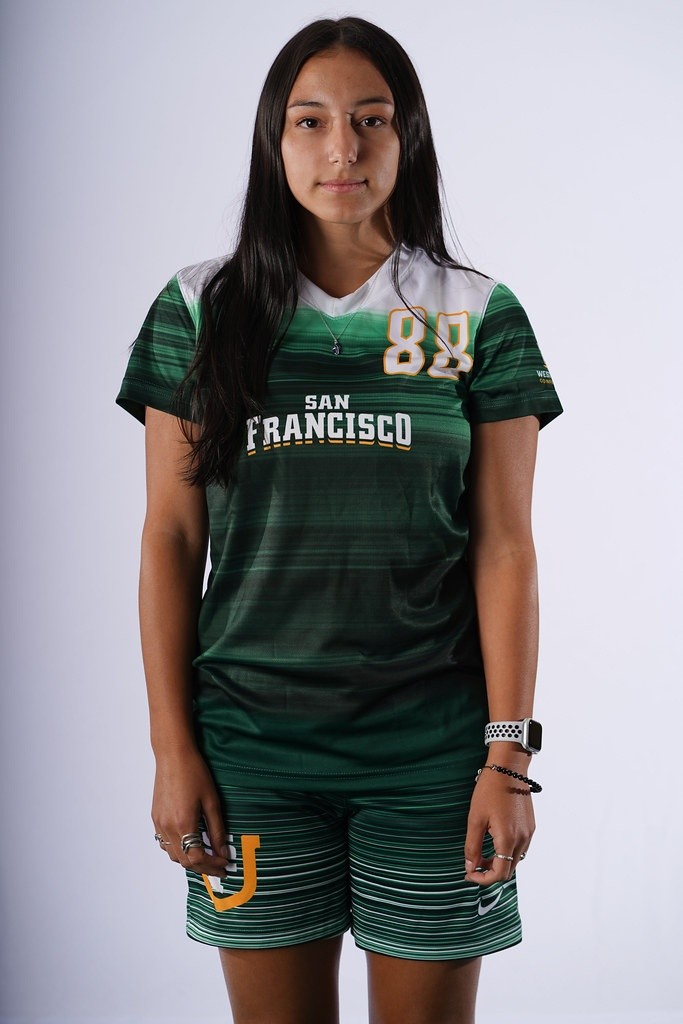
[300,259,387,355]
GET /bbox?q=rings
[518,851,526,861]
[493,854,513,862]
[154,834,162,840]
[181,832,203,854]
[162,841,171,846]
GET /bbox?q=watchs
[485,718,542,754]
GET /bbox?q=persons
[115,17,563,1023]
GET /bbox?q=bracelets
[475,764,542,793]
